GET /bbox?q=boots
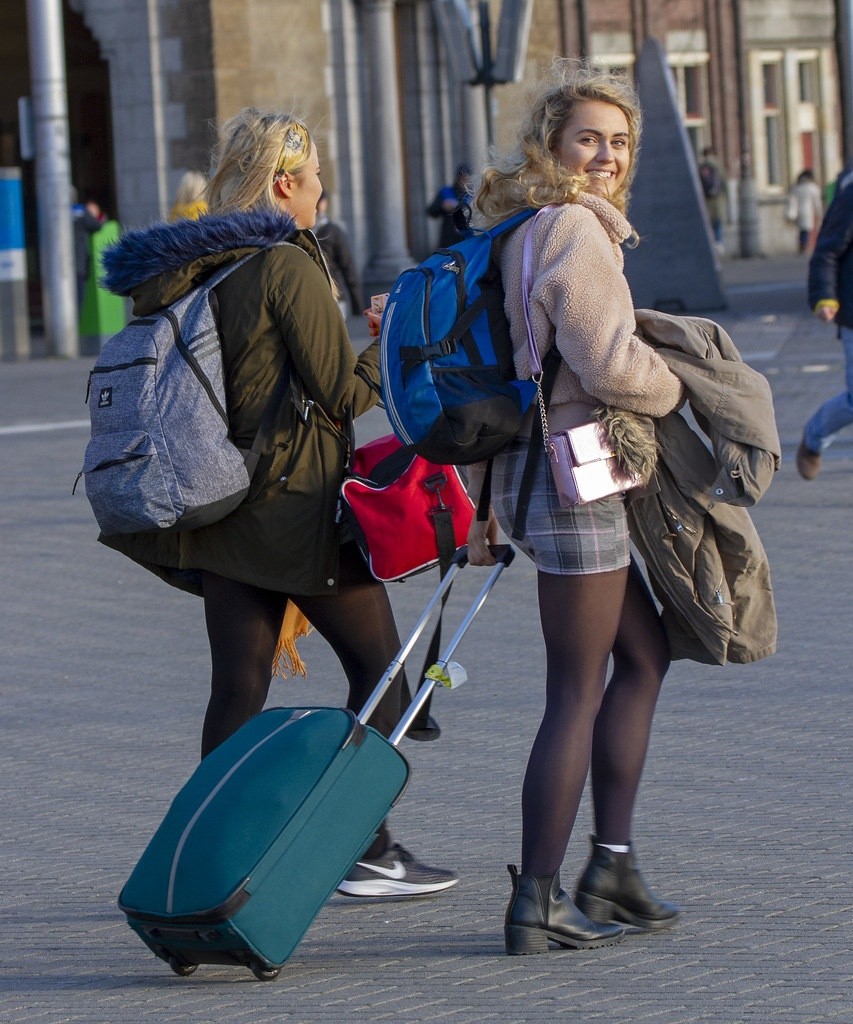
[503,863,626,956]
[576,835,682,929]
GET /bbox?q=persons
[474,81,686,957]
[425,164,475,249]
[701,146,733,256]
[99,109,463,899]
[314,189,364,322]
[794,181,852,480]
[70,187,102,313]
[169,170,209,221]
[787,170,824,251]
[86,201,109,226]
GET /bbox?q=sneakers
[336,843,460,897]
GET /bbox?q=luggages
[118,543,516,981]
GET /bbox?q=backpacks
[698,160,721,198]
[73,242,311,542]
[379,194,540,466]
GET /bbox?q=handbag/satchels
[784,188,799,222]
[334,434,477,581]
[542,421,652,509]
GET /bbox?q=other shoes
[798,425,820,479]
